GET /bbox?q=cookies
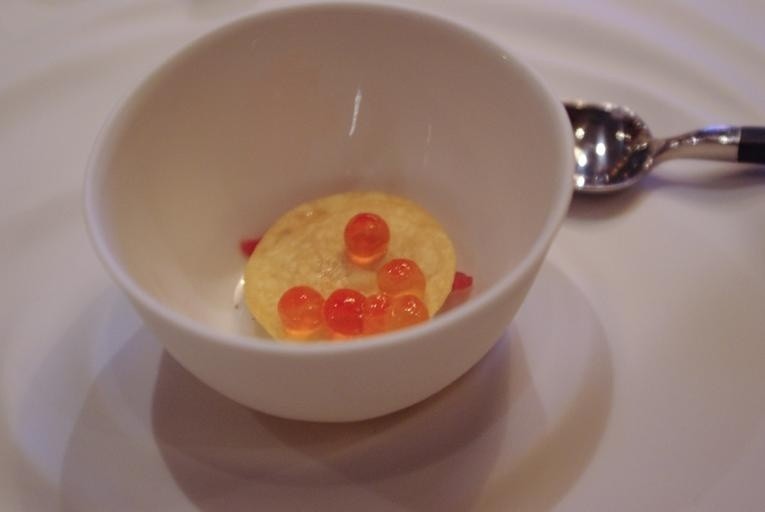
[243,189,458,341]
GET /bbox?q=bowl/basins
[82,0,574,425]
[150,259,614,512]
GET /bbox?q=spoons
[565,96,763,194]
